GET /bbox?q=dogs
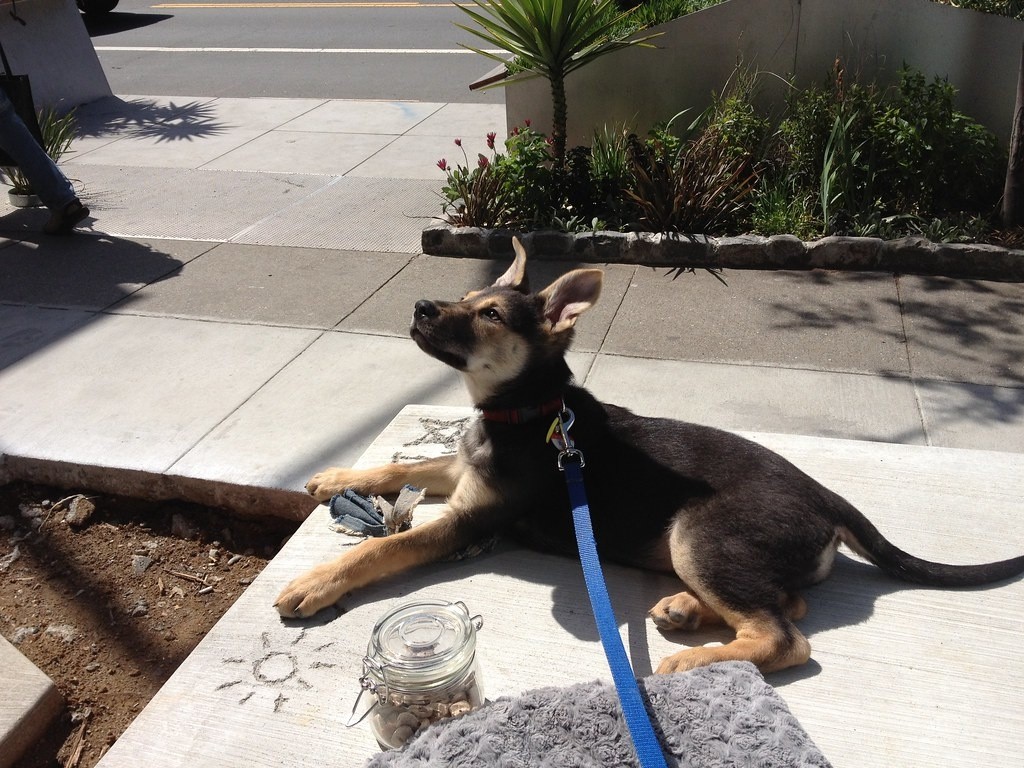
[271,236,1023,676]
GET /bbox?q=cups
[343,599,485,750]
